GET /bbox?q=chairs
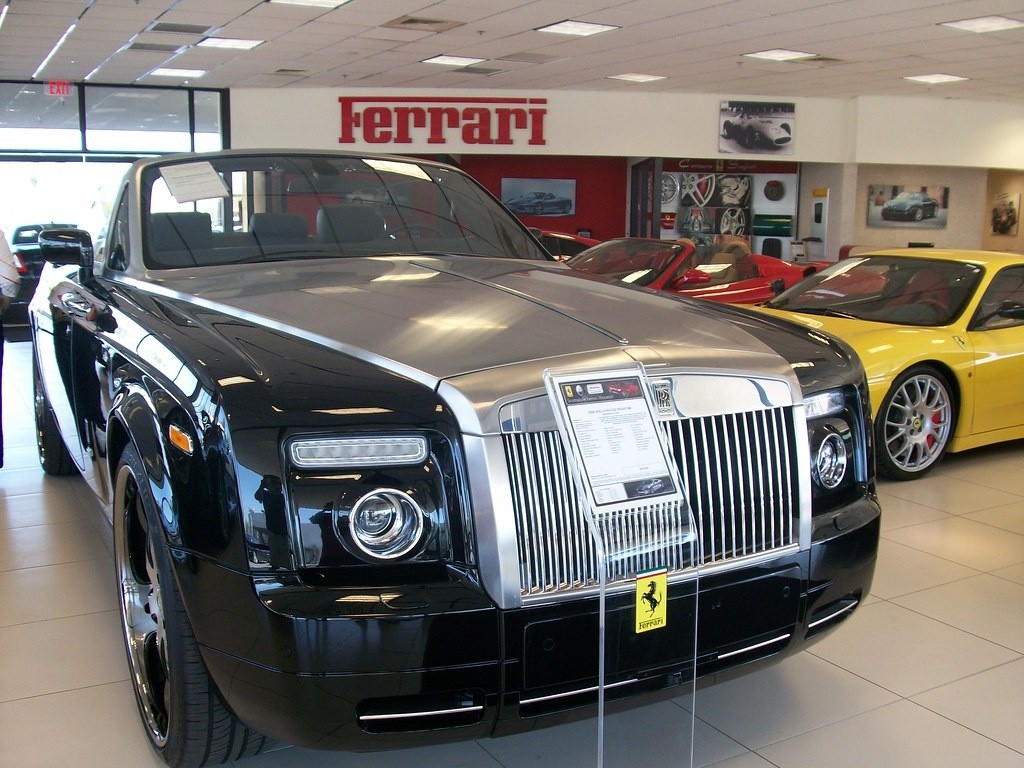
[671,239,697,279]
[151,212,212,255]
[881,267,952,323]
[706,242,759,285]
[211,212,319,248]
[317,203,381,243]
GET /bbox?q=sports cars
[568,235,893,315]
[723,111,792,149]
[504,191,573,215]
[880,192,939,221]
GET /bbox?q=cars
[25,150,883,768]
[530,225,600,257]
[11,223,81,292]
[706,251,1024,480]
